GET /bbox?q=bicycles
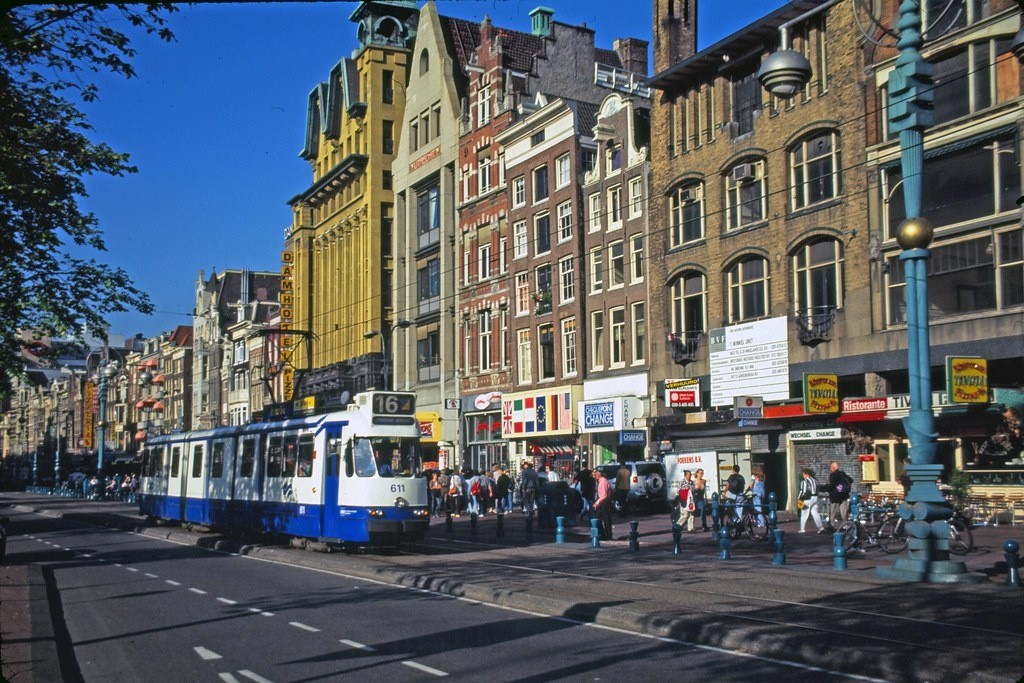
[718,487,976,558]
[86,484,137,503]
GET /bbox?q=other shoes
[798,530,805,533]
[817,526,825,534]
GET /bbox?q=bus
[138,390,430,553]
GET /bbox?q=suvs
[569,458,666,516]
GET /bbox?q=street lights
[85,334,124,472]
[363,319,410,390]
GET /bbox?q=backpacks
[470,478,483,495]
[836,472,851,493]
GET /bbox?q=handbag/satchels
[450,487,457,495]
[801,490,810,499]
[729,474,740,488]
[686,489,695,511]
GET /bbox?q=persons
[796,470,824,533]
[55,472,141,502]
[692,469,709,532]
[744,465,766,527]
[898,458,913,495]
[246,448,417,482]
[673,470,698,534]
[723,465,746,527]
[421,460,631,540]
[819,462,854,530]
